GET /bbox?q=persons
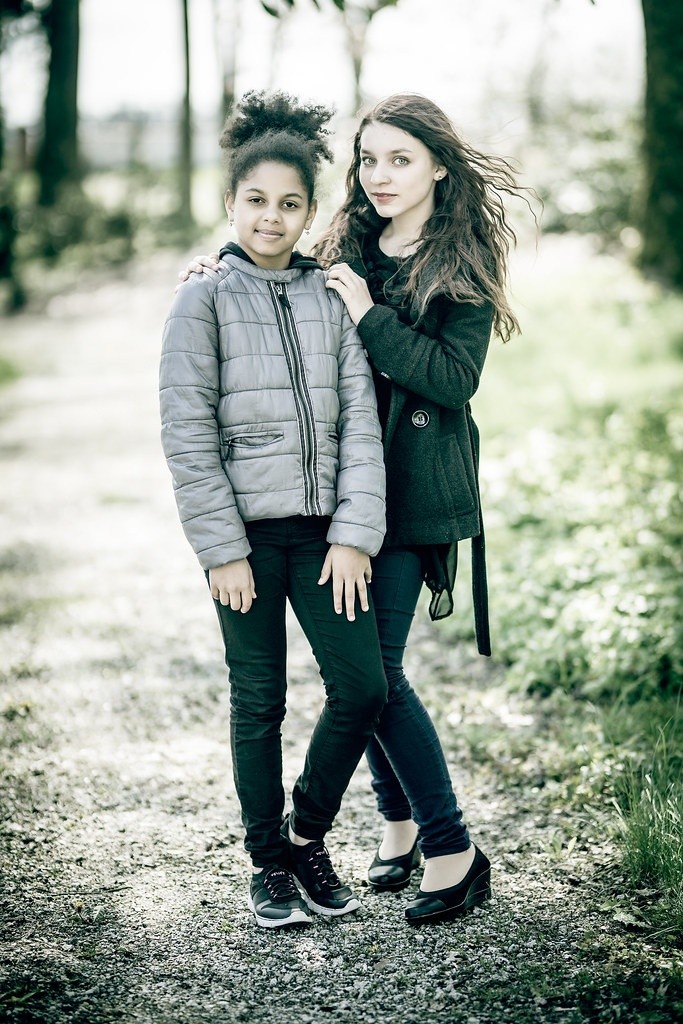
[177,94,522,923]
[160,90,386,929]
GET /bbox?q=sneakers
[279,811,362,917]
[249,862,313,929]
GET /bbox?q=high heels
[366,834,422,887]
[402,840,492,923]
